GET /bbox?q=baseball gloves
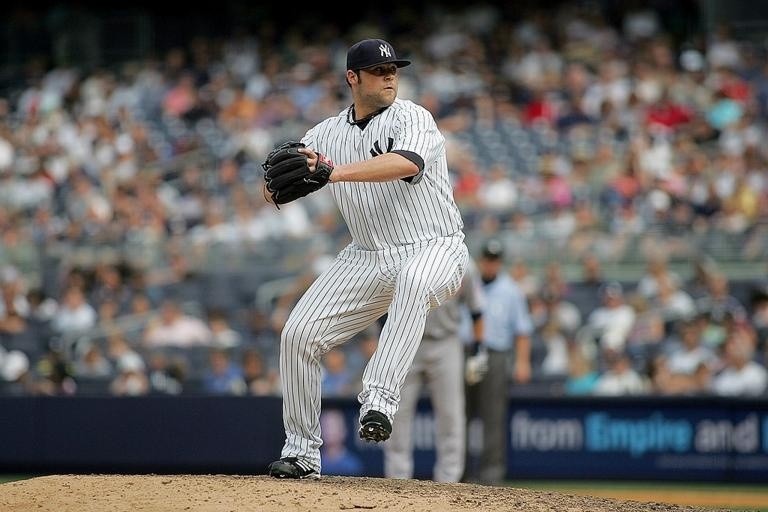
[261,140,334,210]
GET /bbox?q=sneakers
[269,457,320,480]
[357,410,392,442]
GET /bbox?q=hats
[347,38,410,70]
[480,238,504,259]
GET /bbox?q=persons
[318,411,367,477]
[385,240,533,483]
[1,0,767,397]
[259,39,468,480]
